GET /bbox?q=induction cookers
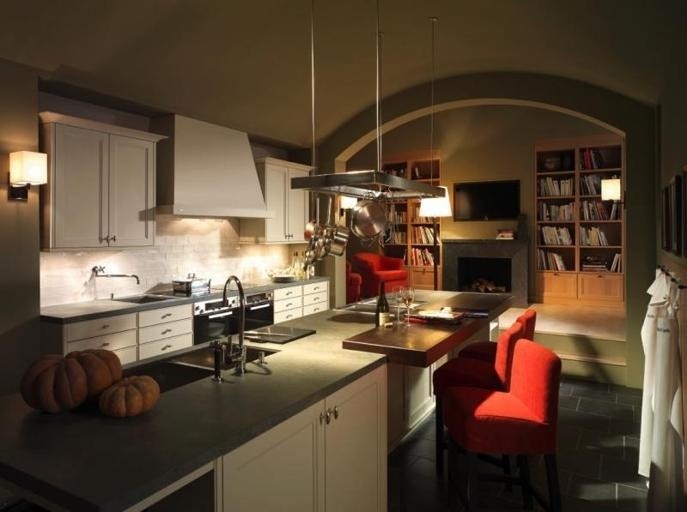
[145,284,275,306]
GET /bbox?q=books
[580,223,610,246]
[539,176,574,196]
[403,309,465,322]
[402,248,434,266]
[413,226,440,245]
[580,174,617,195]
[402,317,462,326]
[537,200,575,221]
[582,252,622,273]
[541,226,573,246]
[537,249,568,271]
[579,200,622,220]
[389,232,407,244]
[387,205,407,223]
[413,207,433,223]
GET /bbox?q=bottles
[375,280,389,328]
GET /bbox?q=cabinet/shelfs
[59,307,138,364]
[138,302,194,359]
[387,361,435,458]
[578,135,623,303]
[378,162,408,266]
[240,157,309,244]
[408,159,441,291]
[272,285,303,324]
[221,363,390,512]
[303,280,329,316]
[535,138,578,303]
[39,111,167,254]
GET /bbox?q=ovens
[194,302,275,345]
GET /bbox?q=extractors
[155,114,271,220]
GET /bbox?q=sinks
[121,360,215,395]
[112,294,189,305]
[166,340,283,371]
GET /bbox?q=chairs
[442,338,563,512]
[346,258,362,303]
[433,322,524,481]
[460,309,536,355]
[353,251,408,296]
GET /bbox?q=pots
[301,192,320,272]
[172,272,212,297]
[314,192,334,261]
[349,191,387,240]
[329,193,350,257]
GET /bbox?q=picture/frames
[668,175,681,256]
[661,186,670,252]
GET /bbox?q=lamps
[419,186,452,290]
[601,178,626,210]
[7,151,48,200]
[339,197,360,218]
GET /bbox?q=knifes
[244,330,288,336]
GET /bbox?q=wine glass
[392,286,404,324]
[401,287,416,326]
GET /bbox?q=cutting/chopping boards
[237,324,316,345]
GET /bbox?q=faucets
[223,275,249,367]
[92,266,140,285]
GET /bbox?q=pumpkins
[99,375,160,417]
[20,355,89,414]
[65,348,124,405]
